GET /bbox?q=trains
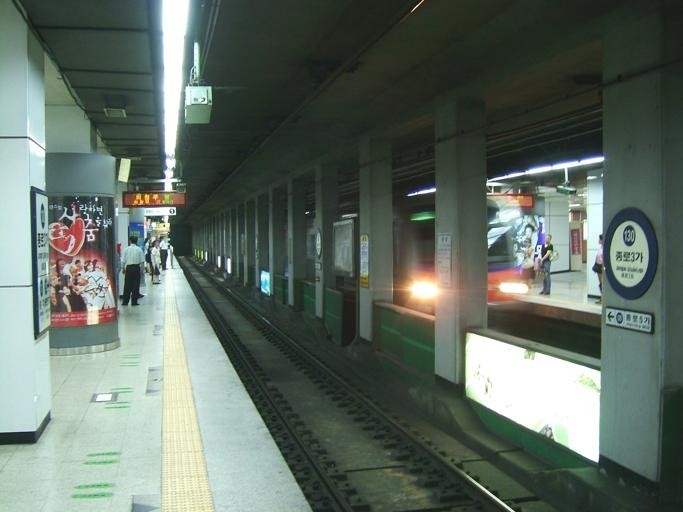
[306,198,527,315]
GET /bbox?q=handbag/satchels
[521,258,534,270]
[591,263,602,273]
[549,251,560,263]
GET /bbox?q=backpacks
[145,249,152,263]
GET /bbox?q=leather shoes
[121,301,140,306]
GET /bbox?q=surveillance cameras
[184,85,213,124]
[176,183,186,194]
[557,186,578,194]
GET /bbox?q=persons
[116,237,168,305]
[513,224,538,266]
[521,234,553,295]
[593,235,603,303]
[50,258,116,312]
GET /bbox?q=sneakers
[538,291,550,296]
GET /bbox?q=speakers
[118,158,131,184]
